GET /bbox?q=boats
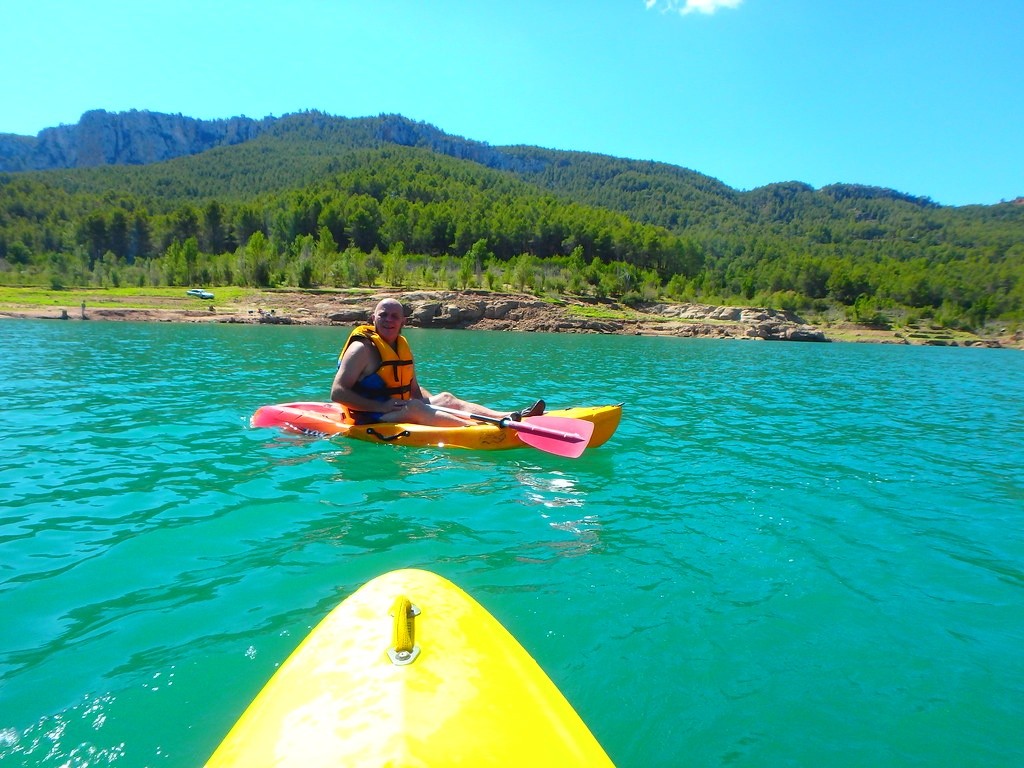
[249,400,625,452]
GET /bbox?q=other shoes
[510,412,522,422]
[521,399,545,416]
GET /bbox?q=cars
[186,288,214,299]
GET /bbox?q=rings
[396,402,397,405]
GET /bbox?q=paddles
[426,401,594,459]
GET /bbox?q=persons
[330,298,546,425]
[82,300,86,316]
